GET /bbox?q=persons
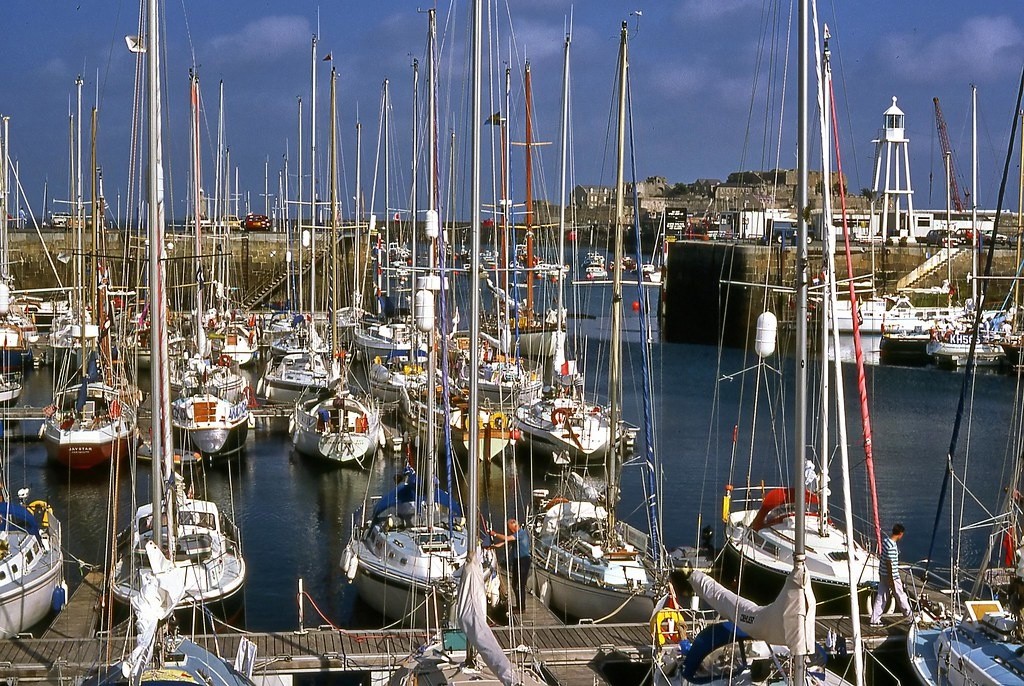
[19,207,28,224]
[1009,536,1024,644]
[965,229,992,243]
[869,524,922,627]
[880,314,1015,346]
[485,519,532,614]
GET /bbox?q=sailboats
[624,1,865,686]
[905,61,1023,686]
[714,1,910,617]
[1,0,704,686]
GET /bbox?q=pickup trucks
[914,229,962,248]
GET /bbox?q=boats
[830,281,1024,370]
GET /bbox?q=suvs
[767,218,815,244]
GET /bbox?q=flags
[216,243,222,251]
[195,266,205,293]
[307,315,311,322]
[483,110,501,126]
[4,337,7,349]
[561,360,579,376]
[25,304,29,312]
[323,54,331,61]
[96,258,111,285]
[810,270,825,286]
[393,213,400,220]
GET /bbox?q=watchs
[494,533,497,538]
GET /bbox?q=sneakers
[871,622,887,627]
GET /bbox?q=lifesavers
[550,408,569,426]
[464,414,484,432]
[544,498,570,512]
[27,500,53,528]
[489,411,507,430]
[218,355,232,368]
[648,607,686,646]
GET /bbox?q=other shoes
[512,606,526,614]
